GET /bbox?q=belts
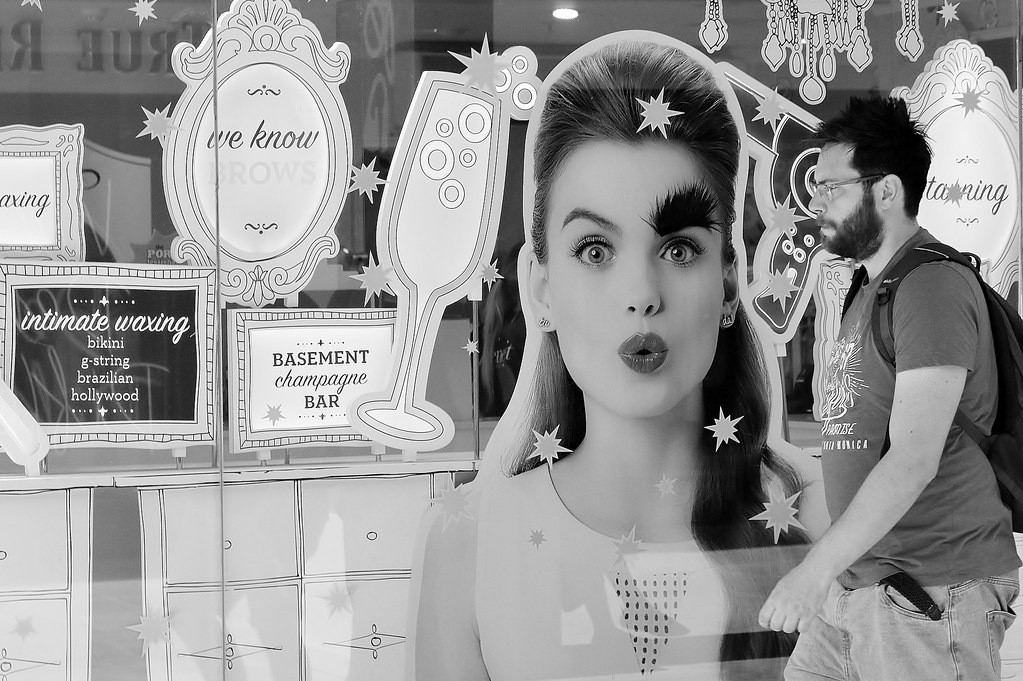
[841,572,942,621]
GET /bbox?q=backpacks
[840,244,1023,534]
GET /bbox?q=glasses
[809,174,881,202]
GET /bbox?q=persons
[759,93,1023,681]
[415,39,831,681]
